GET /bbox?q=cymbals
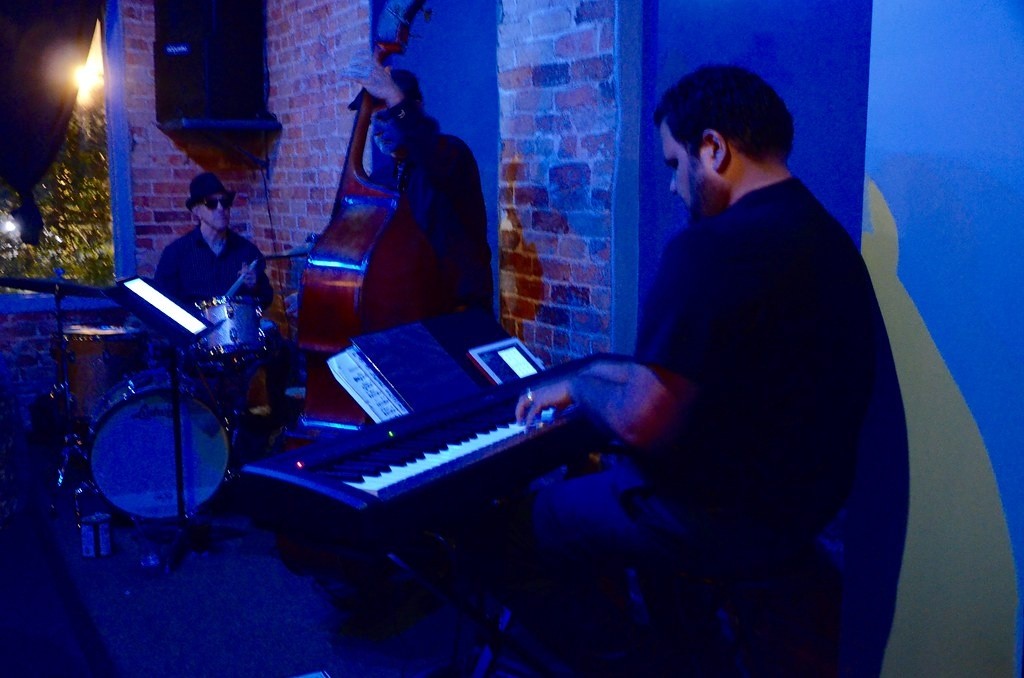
[1,276,106,296]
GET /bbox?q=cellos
[297,0,433,453]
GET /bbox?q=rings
[525,388,536,404]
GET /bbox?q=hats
[185,172,236,210]
[346,67,423,109]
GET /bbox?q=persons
[153,171,303,431]
[514,63,883,678]
[338,53,497,316]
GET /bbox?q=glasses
[197,197,233,209]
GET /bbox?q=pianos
[241,308,636,677]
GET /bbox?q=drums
[64,325,138,395]
[89,371,229,518]
[193,296,266,364]
